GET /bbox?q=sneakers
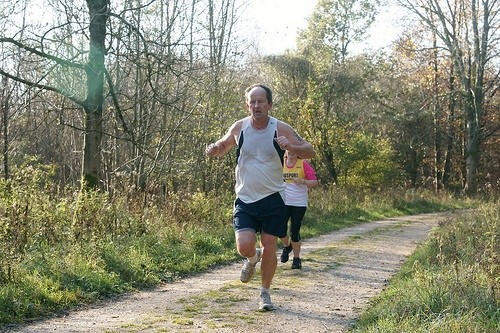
[291,258,301,269]
[258,290,273,311]
[240,249,262,283]
[281,244,292,263]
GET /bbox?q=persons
[275,146,318,268]
[206,84,315,312]
